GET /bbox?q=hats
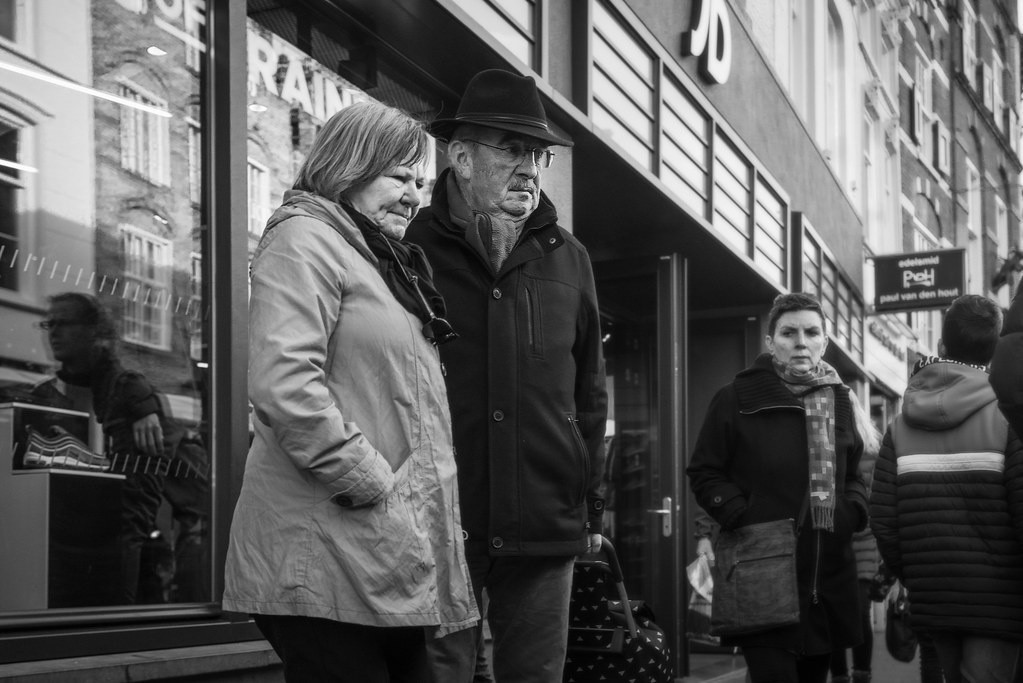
[426,70,574,147]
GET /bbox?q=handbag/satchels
[885,597,918,663]
[709,519,800,637]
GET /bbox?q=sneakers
[22,428,111,471]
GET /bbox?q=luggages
[563,536,672,683]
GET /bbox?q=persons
[868,294,1023,683]
[403,67,609,683]
[831,388,898,683]
[14,291,165,606]
[988,277,1023,440]
[94,262,209,606]
[685,293,870,683]
[224,103,480,683]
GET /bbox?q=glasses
[460,137,556,169]
[415,281,463,370]
[39,318,85,332]
